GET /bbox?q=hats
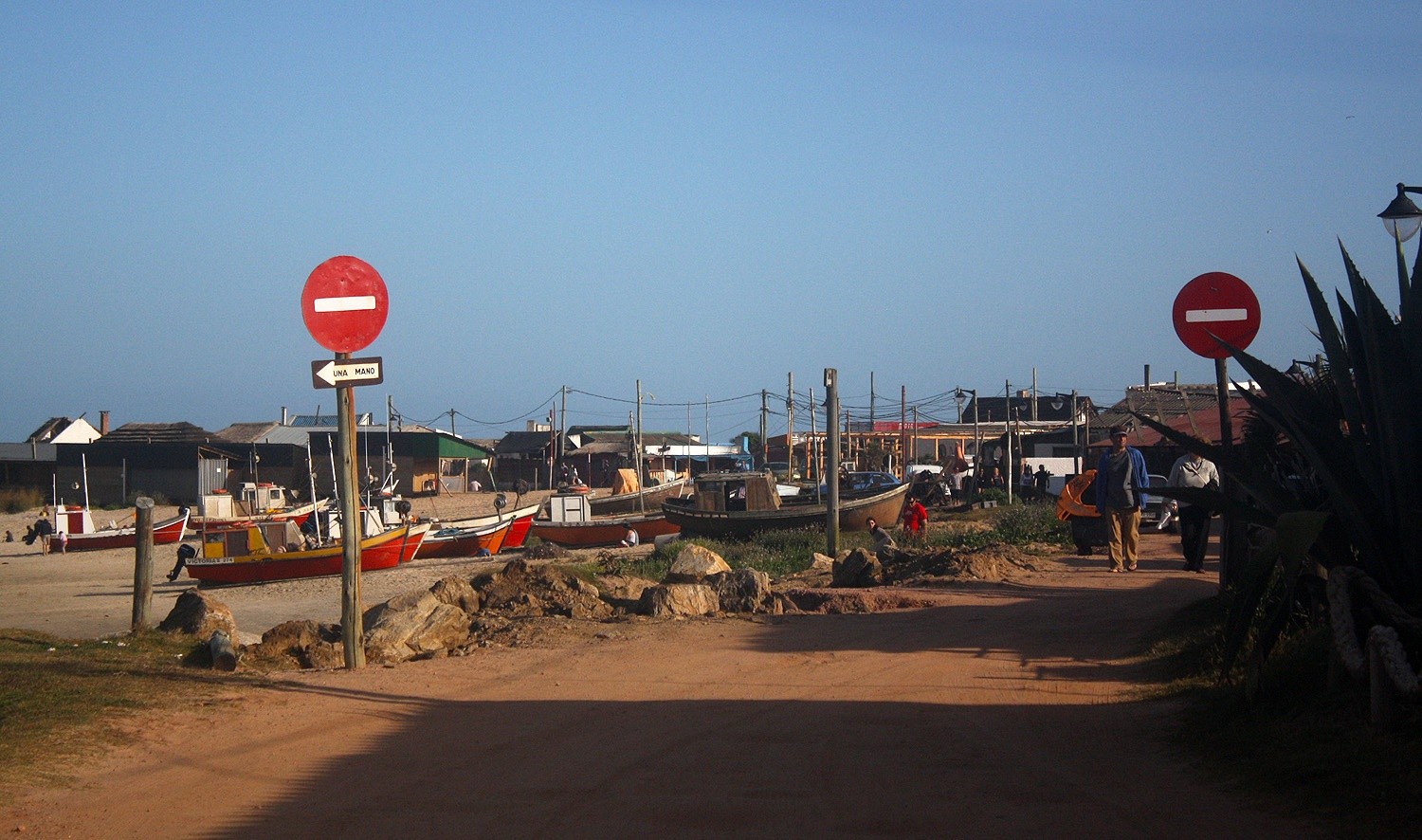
[1110,425,1128,436]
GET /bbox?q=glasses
[907,500,914,504]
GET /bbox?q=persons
[560,462,578,485]
[40,518,52,556]
[623,522,639,547]
[59,531,67,554]
[903,492,928,541]
[1162,435,1219,573]
[1034,464,1050,492]
[1022,466,1032,484]
[866,517,891,544]
[949,467,963,506]
[26,525,33,535]
[991,468,1004,487]
[1095,425,1149,572]
[5,531,12,542]
[977,469,985,492]
[469,479,482,491]
[179,500,190,515]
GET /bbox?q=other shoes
[1196,568,1205,573]
[1183,562,1192,570]
[1128,564,1137,571]
[1108,568,1122,572]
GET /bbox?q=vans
[904,464,952,500]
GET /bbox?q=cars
[811,471,903,496]
[755,461,802,481]
[1141,475,1180,528]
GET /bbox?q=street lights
[955,388,980,473]
[1051,389,1079,476]
[1009,398,1028,457]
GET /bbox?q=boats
[661,471,911,539]
[530,378,687,551]
[184,390,540,591]
[43,452,190,551]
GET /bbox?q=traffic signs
[312,355,384,388]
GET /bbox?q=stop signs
[1172,271,1263,359]
[301,256,389,354]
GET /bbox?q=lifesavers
[212,489,227,495]
[233,521,252,527]
[271,517,286,522]
[567,486,588,494]
[259,483,272,486]
[64,506,81,510]
